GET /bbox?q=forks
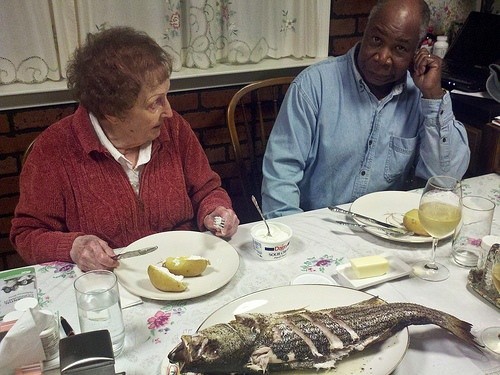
[323,217,414,238]
[213,215,226,229]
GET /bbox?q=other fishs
[168,296,486,375]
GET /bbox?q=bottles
[39,310,62,363]
[418,27,435,50]
[433,35,449,59]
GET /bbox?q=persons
[259,0,471,219]
[9,24,240,272]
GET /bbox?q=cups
[450,195,496,269]
[73,269,125,359]
[476,235,500,271]
[4,297,39,322]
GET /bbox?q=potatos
[165,257,208,276]
[147,265,187,292]
[403,208,433,236]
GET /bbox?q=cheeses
[349,255,389,279]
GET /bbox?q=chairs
[227,75,297,223]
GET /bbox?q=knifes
[112,245,158,261]
[328,205,398,229]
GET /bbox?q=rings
[428,54,431,58]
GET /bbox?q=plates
[336,252,412,290]
[349,190,455,243]
[114,230,240,300]
[196,283,409,375]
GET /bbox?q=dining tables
[0,171,500,375]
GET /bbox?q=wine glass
[411,175,464,282]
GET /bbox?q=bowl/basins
[249,221,294,261]
